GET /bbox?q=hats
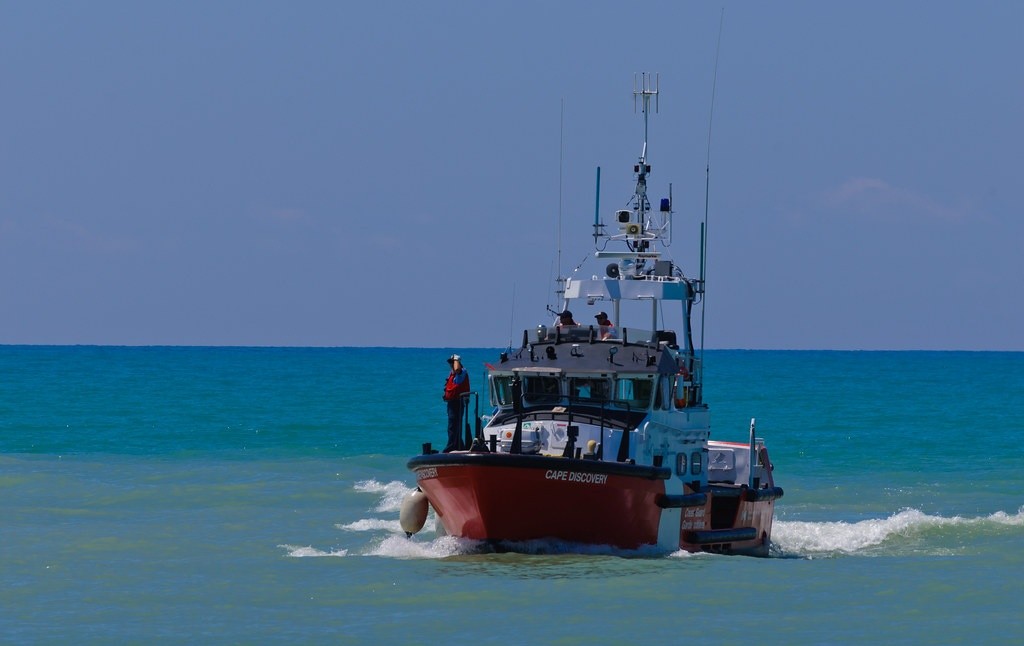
[447,355,454,363]
[557,311,572,317]
[595,312,607,320]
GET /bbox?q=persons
[557,311,577,326]
[594,311,619,341]
[443,354,470,453]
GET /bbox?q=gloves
[454,354,460,361]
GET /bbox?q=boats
[404,4,784,558]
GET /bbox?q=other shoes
[442,443,454,452]
[455,440,464,450]
